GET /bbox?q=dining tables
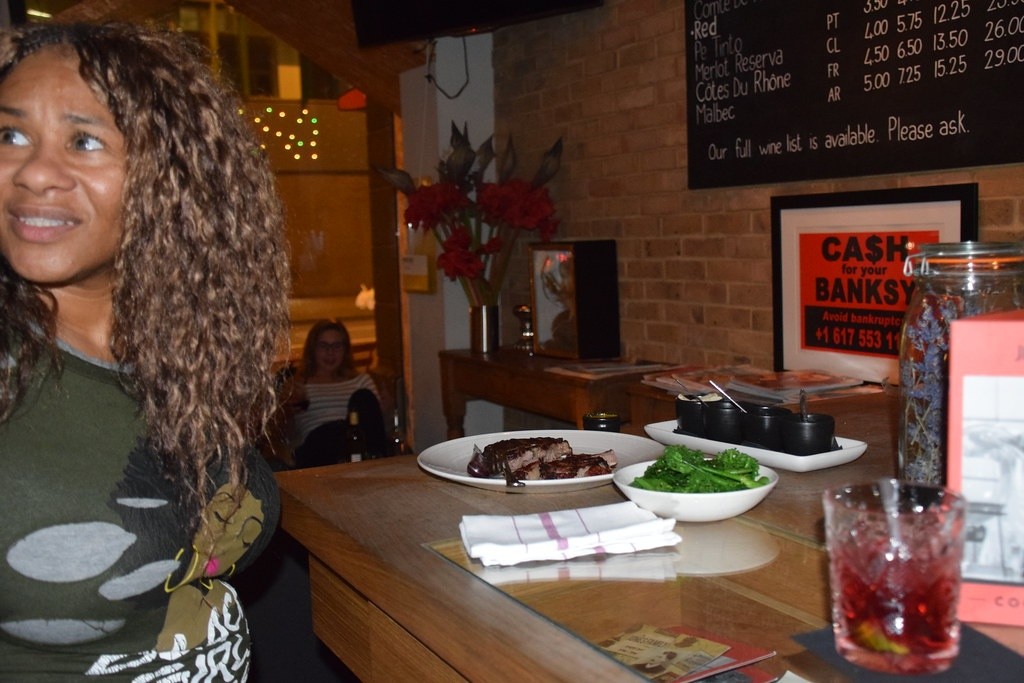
[275,402,1024,683]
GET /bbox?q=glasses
[315,343,345,352]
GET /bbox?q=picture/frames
[769,177,977,385]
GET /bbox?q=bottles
[898,241,1022,484]
[342,411,368,464]
[387,409,406,456]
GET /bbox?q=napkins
[455,499,682,564]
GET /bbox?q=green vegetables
[626,445,771,494]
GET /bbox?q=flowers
[370,122,566,308]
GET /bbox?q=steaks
[480,437,618,481]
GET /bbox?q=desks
[436,346,681,440]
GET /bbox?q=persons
[281,316,398,470]
[0,17,292,683]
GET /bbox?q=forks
[488,449,525,488]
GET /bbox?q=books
[640,362,772,396]
[724,368,865,400]
[542,356,685,381]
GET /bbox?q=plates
[417,429,667,494]
[645,419,867,473]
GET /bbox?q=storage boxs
[527,242,619,361]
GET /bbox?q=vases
[471,305,498,353]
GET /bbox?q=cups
[781,411,835,458]
[743,403,792,453]
[675,392,724,437]
[702,398,747,445]
[821,481,966,676]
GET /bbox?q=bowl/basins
[613,458,779,523]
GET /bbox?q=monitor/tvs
[352,0,606,51]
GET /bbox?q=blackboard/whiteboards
[685,0,1024,190]
